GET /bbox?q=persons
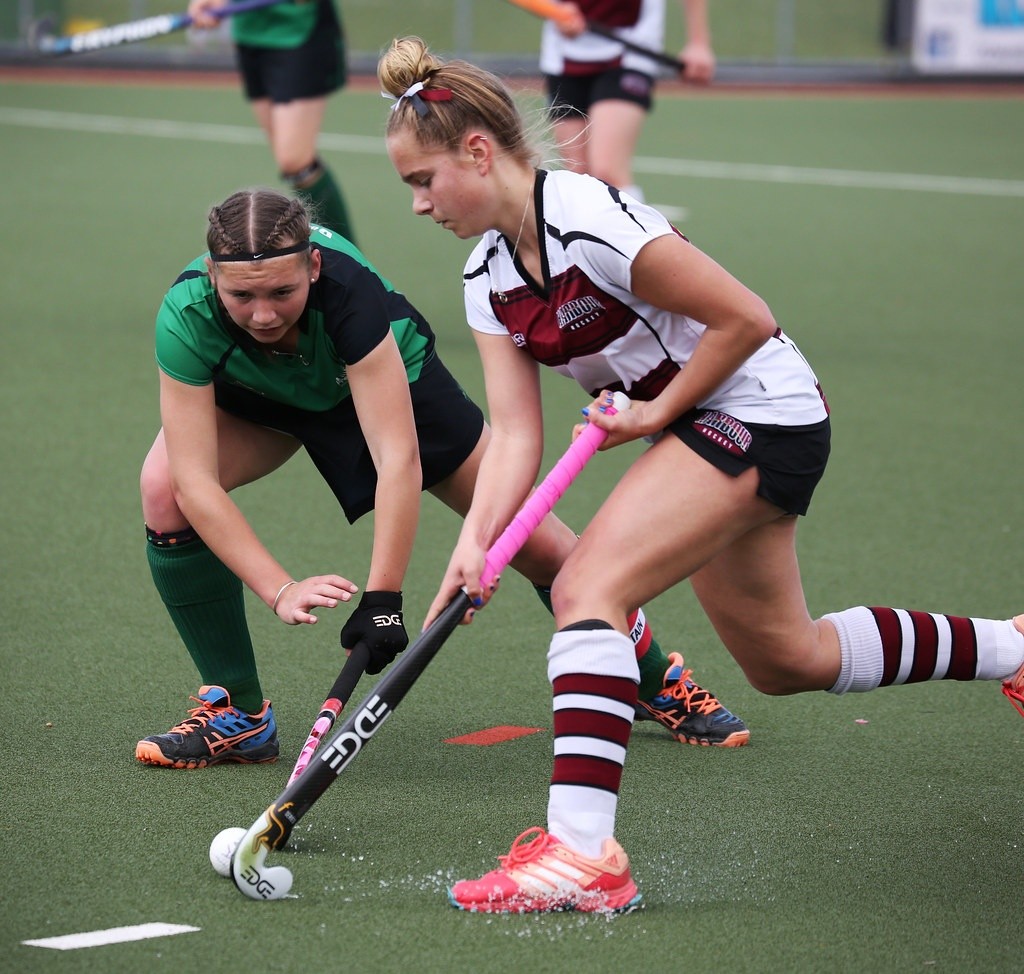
[512,0,719,202]
[381,33,1024,913]
[136,191,750,771]
[190,1,356,245]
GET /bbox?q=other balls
[209,827,247,876]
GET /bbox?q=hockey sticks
[583,24,688,76]
[34,0,277,57]
[229,388,632,902]
[274,643,365,851]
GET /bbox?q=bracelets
[272,581,298,615]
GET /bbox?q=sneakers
[448,828,642,913]
[135,684,280,769]
[635,652,750,749]
[999,614,1024,716]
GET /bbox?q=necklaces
[495,181,532,303]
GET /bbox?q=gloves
[341,589,408,676]
